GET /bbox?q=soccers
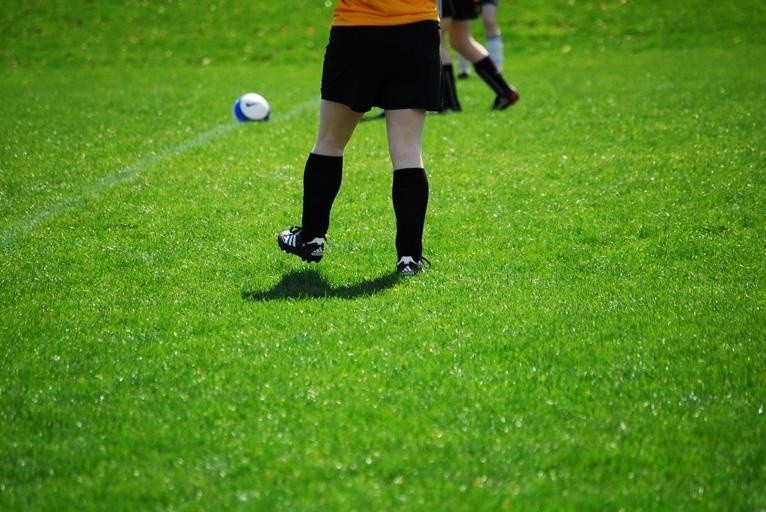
[235,93,270,121]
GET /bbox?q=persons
[275,1,443,277]
[430,1,520,114]
[455,1,506,80]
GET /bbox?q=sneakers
[278,226,325,263]
[492,88,518,110]
[396,256,425,275]
